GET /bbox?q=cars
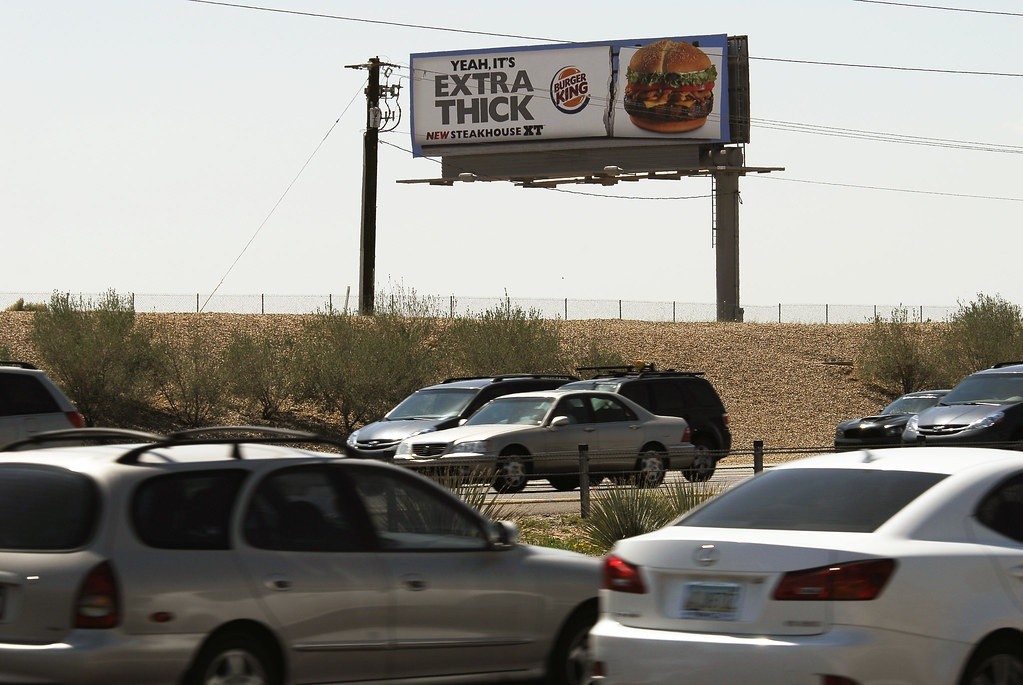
[392,389,695,494]
[833,389,955,450]
[588,449,1023,685]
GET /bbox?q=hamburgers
[623,39,718,133]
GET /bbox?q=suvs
[1,360,85,450]
[345,373,580,465]
[556,363,732,484]
[0,426,606,685]
[898,360,1023,451]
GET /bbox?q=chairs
[186,490,229,518]
[281,500,351,548]
